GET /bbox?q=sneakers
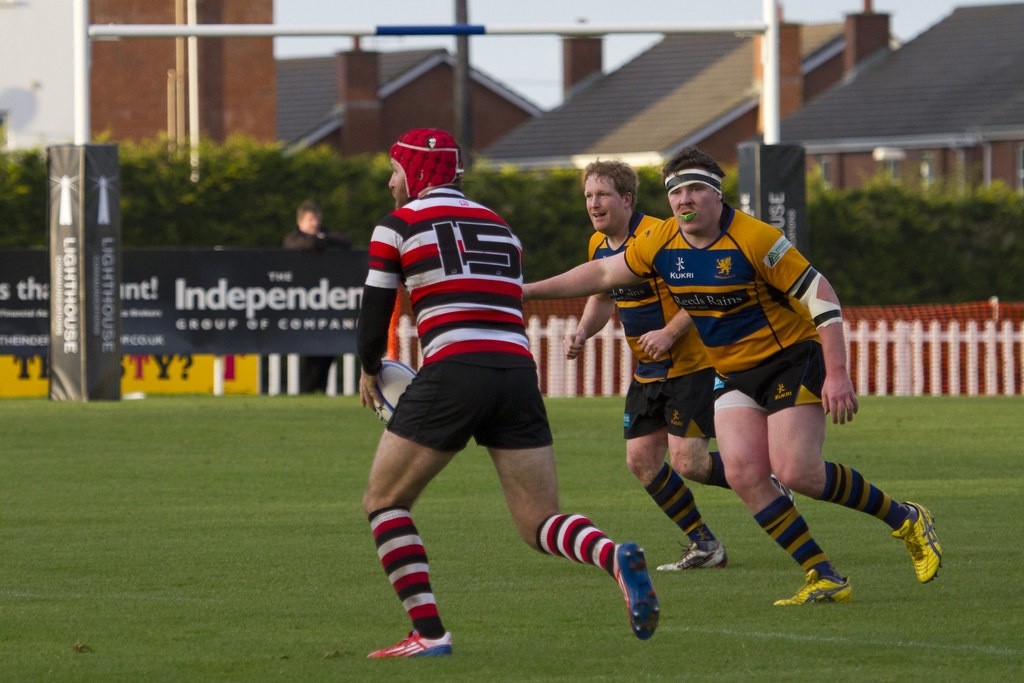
[367,630,453,659]
[773,569,855,608]
[770,474,797,511]
[656,538,728,571]
[613,541,660,640]
[892,501,943,584]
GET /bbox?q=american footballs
[369,359,418,426]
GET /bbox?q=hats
[389,127,464,199]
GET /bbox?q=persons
[564,158,796,572]
[276,201,350,395]
[522,145,942,606]
[355,127,661,659]
